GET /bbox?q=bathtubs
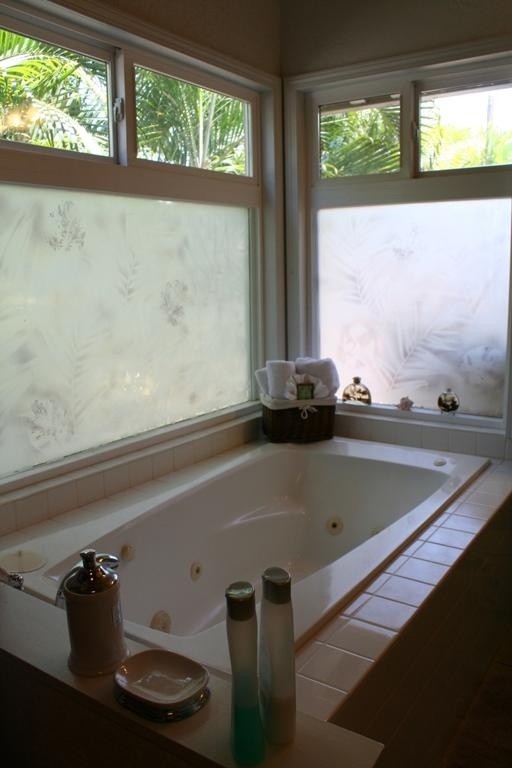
[0,439,492,677]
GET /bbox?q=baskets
[260,394,337,444]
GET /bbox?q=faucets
[55,553,119,610]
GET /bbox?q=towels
[255,357,340,400]
